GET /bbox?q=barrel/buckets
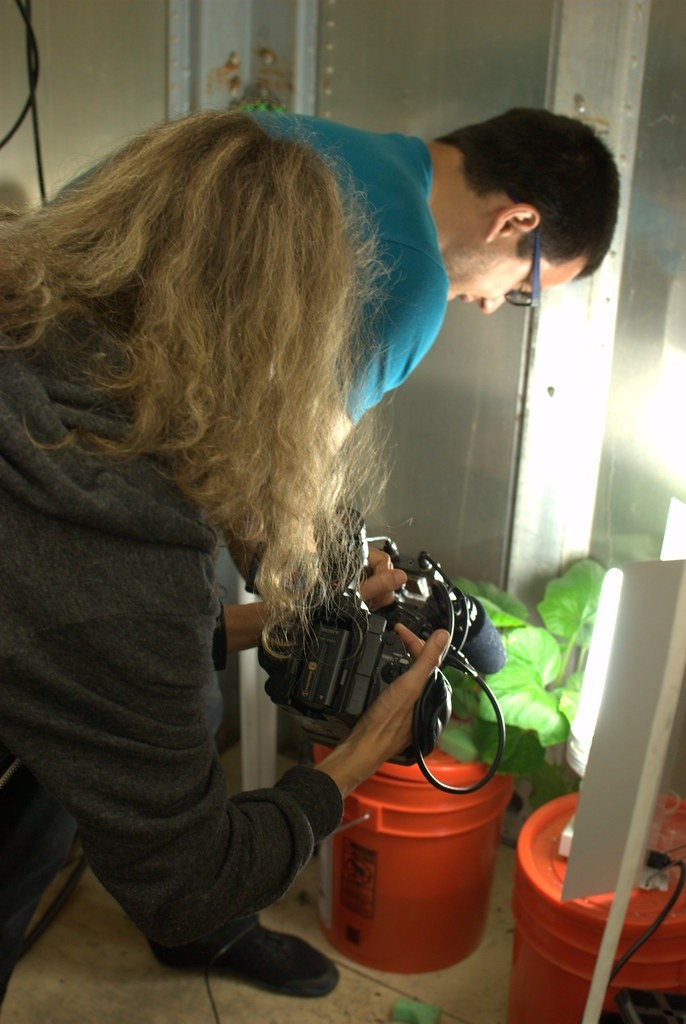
[309,733,517,972]
[506,787,686,1024]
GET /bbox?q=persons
[0,113,452,994]
[24,109,621,1001]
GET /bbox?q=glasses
[504,227,540,308]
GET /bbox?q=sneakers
[146,915,344,1000]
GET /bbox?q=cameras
[261,505,506,767]
